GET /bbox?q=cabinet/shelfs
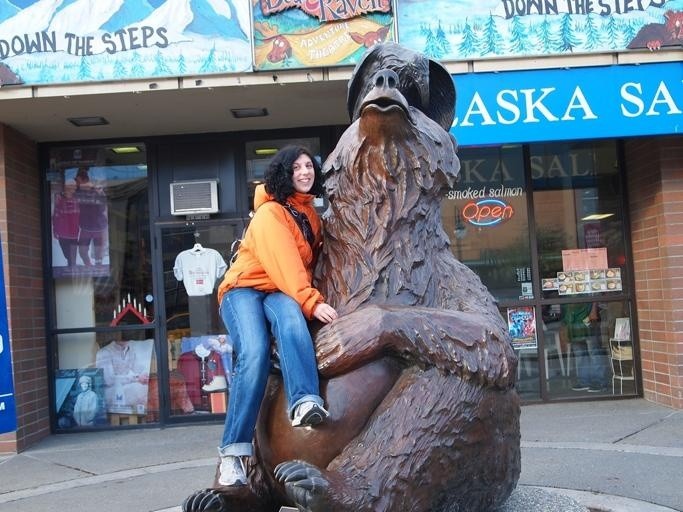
[609,338,635,396]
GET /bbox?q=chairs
[517,318,566,380]
[567,340,592,379]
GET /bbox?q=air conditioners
[169,178,221,221]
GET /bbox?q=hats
[76,167,89,177]
[77,375,91,386]
[63,179,77,190]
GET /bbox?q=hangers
[191,232,203,252]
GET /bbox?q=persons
[216,144,337,488]
[96,340,149,407]
[53,179,81,265]
[541,319,560,377]
[73,376,98,427]
[54,169,107,264]
[563,301,608,393]
[217,335,232,383]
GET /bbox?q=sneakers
[290,401,330,428]
[201,376,227,392]
[573,382,589,391]
[218,455,248,487]
[587,386,607,392]
